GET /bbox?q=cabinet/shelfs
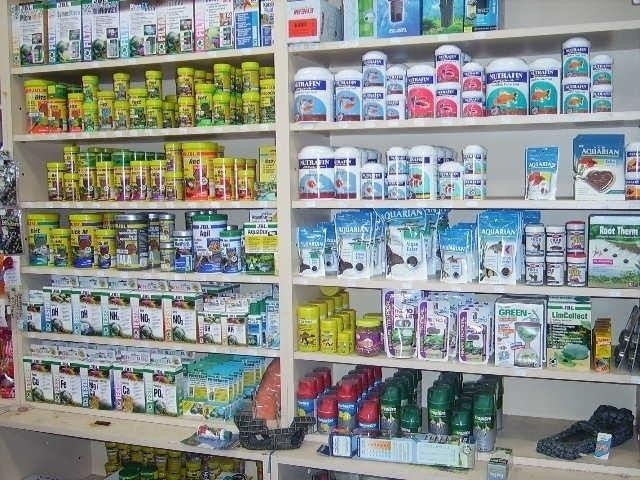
[103,442,245,480]
[625,141,640,200]
[523,222,587,288]
[296,362,505,458]
[295,285,356,356]
[297,142,488,201]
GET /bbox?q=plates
[2,0,288,434]
[288,1,640,480]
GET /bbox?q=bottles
[296,362,505,458]
[46,142,276,200]
[625,141,640,200]
[295,285,356,356]
[292,37,613,122]
[103,442,245,480]
[26,210,275,276]
[297,142,488,201]
[23,61,276,131]
[523,222,587,288]
